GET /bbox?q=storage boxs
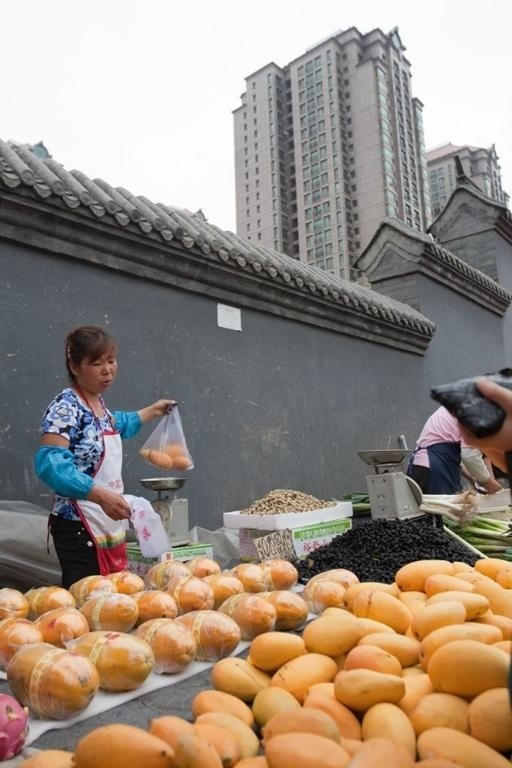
[121,539,215,580]
[236,513,354,567]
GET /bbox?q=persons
[33,322,180,592]
[406,367,512,496]
[481,454,510,489]
[456,377,512,502]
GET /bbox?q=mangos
[15,560,511,768]
[139,444,191,470]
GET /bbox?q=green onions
[339,491,512,561]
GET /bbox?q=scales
[358,449,427,521]
[136,477,192,547]
